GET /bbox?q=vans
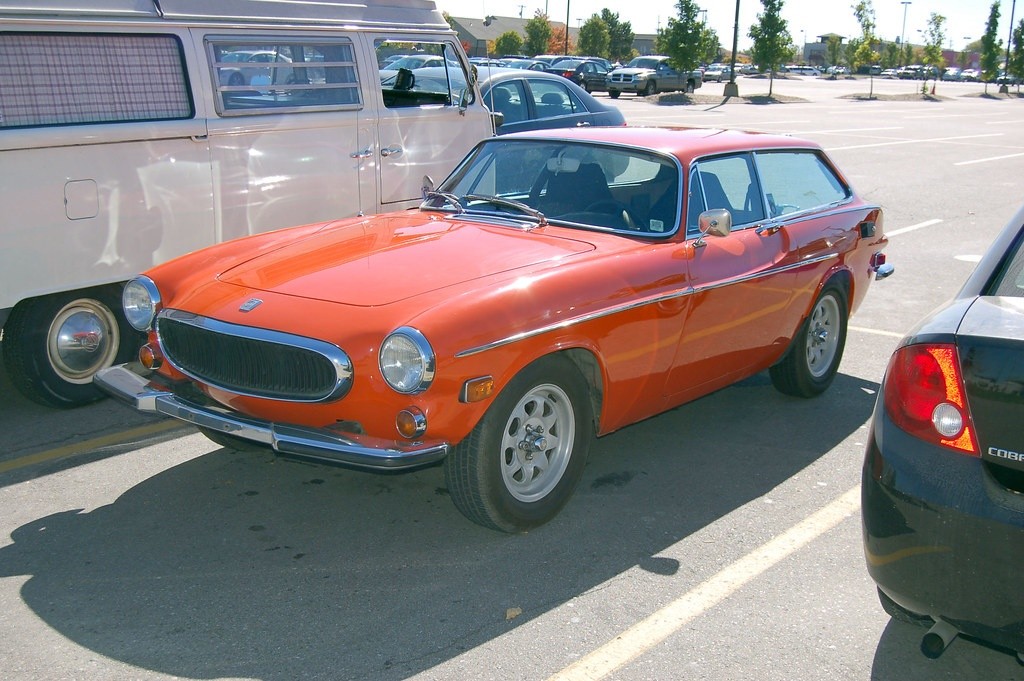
[787,65,822,77]
[1,0,505,412]
[869,65,883,75]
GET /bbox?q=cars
[702,65,738,84]
[882,64,1017,84]
[385,54,792,73]
[382,54,458,71]
[545,58,615,95]
[738,64,760,75]
[861,197,1024,660]
[380,66,627,136]
[824,65,854,75]
[91,122,897,534]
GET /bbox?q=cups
[393,68,415,89]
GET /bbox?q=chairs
[641,170,734,234]
[542,163,618,224]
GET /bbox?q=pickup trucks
[605,55,703,98]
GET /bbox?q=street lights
[800,30,807,75]
[899,2,913,69]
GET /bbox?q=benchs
[489,87,571,123]
